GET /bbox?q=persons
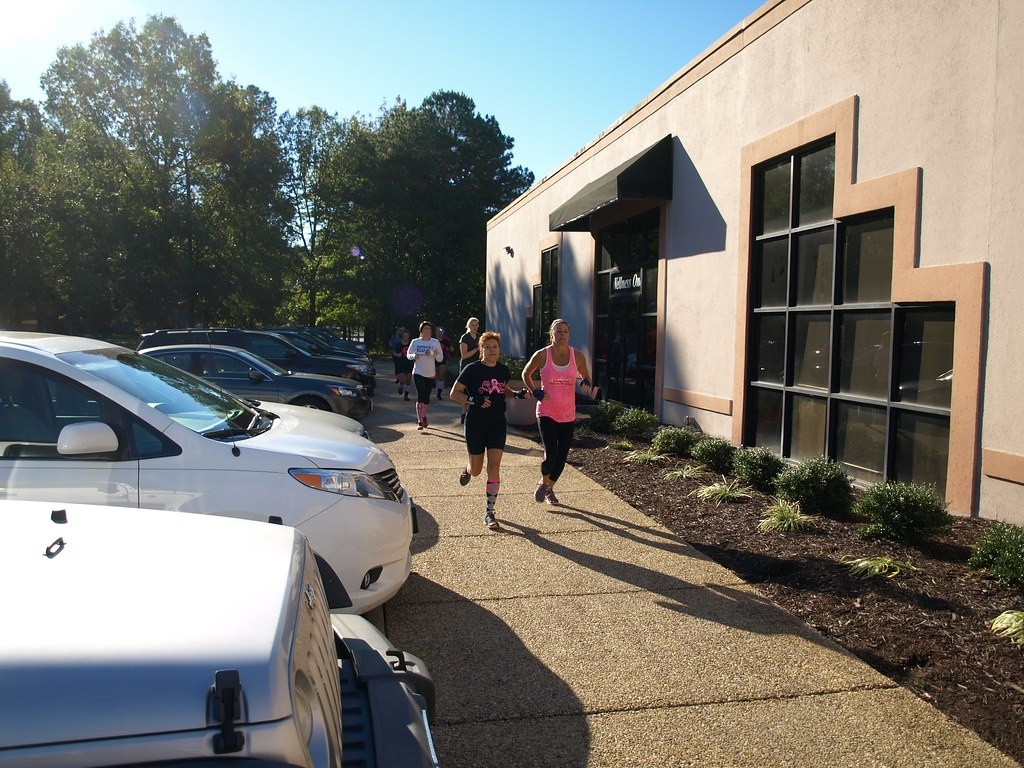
[388,323,454,401]
[406,320,445,430]
[871,332,890,402]
[449,330,533,530]
[459,317,483,425]
[520,318,592,505]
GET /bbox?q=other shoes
[423,417,428,428]
[404,391,410,401]
[398,387,404,395]
[417,422,423,430]
[534,480,558,504]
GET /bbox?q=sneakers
[460,468,471,486]
[484,511,499,528]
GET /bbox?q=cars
[137,344,374,424]
[136,326,377,396]
[0,497,443,768]
[0,331,420,621]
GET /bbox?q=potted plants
[501,356,541,426]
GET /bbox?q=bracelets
[475,347,478,351]
